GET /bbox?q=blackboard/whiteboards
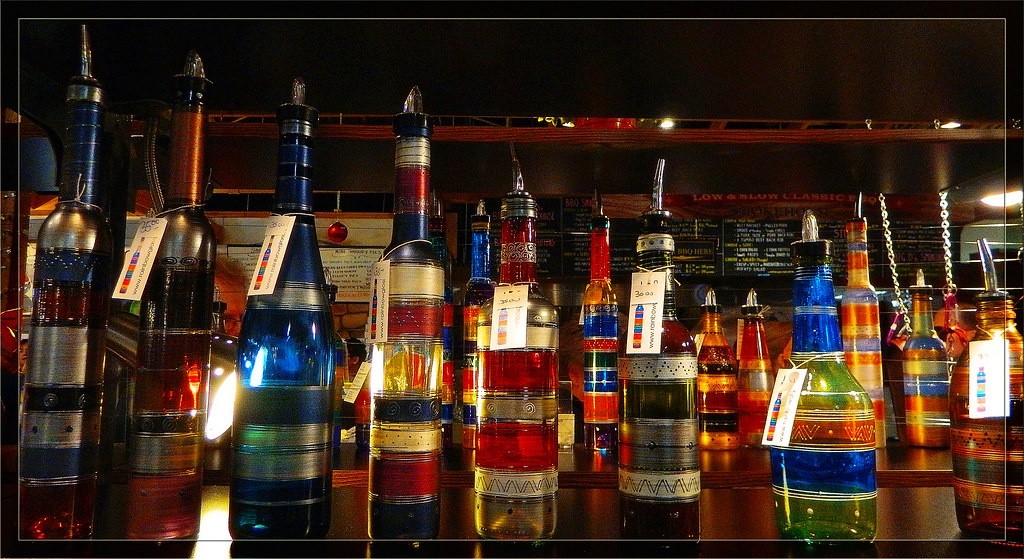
[464,194,972,280]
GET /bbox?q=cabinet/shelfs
[0,0,1024,559]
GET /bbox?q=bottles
[770,212,878,549]
[129,52,216,476]
[0,155,738,521]
[840,192,889,449]
[736,289,779,450]
[16,24,114,497]
[361,92,444,504]
[231,77,333,537]
[950,238,1024,541]
[903,270,951,449]
